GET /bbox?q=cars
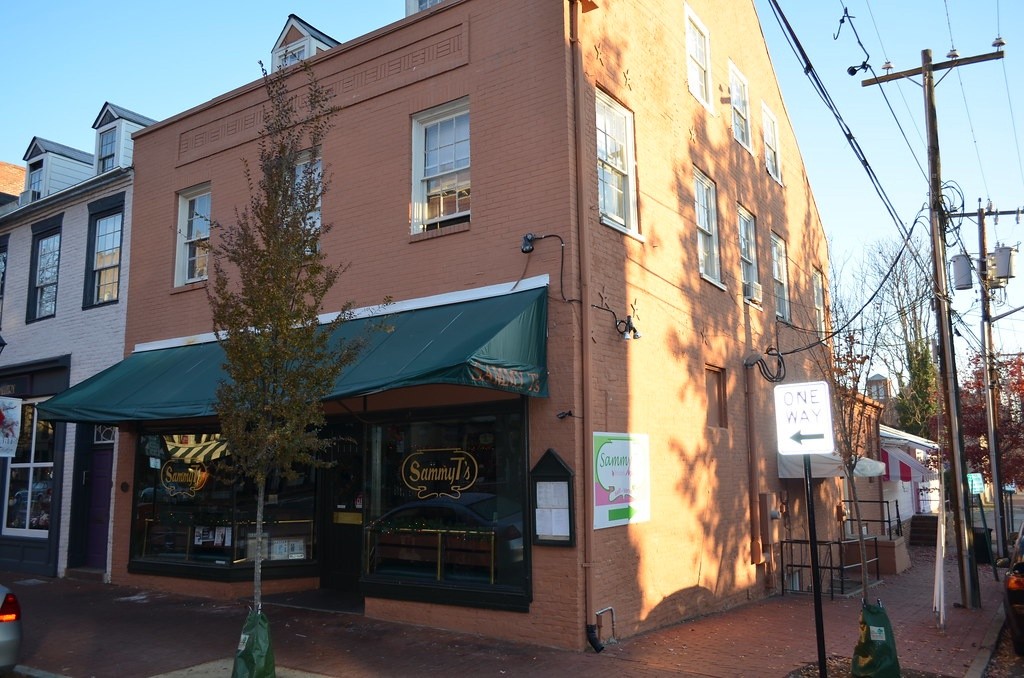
[139,474,233,505]
[996,520,1024,618]
[367,490,524,566]
[0,580,23,678]
[14,478,53,513]
[1001,481,1017,494]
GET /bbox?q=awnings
[34,271,550,423]
[880,445,939,483]
[777,451,887,479]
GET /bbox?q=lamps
[617,315,642,341]
[521,233,534,254]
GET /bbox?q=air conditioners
[18,189,38,207]
[742,279,763,305]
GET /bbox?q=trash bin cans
[972,527,993,564]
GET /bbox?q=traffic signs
[775,381,835,456]
